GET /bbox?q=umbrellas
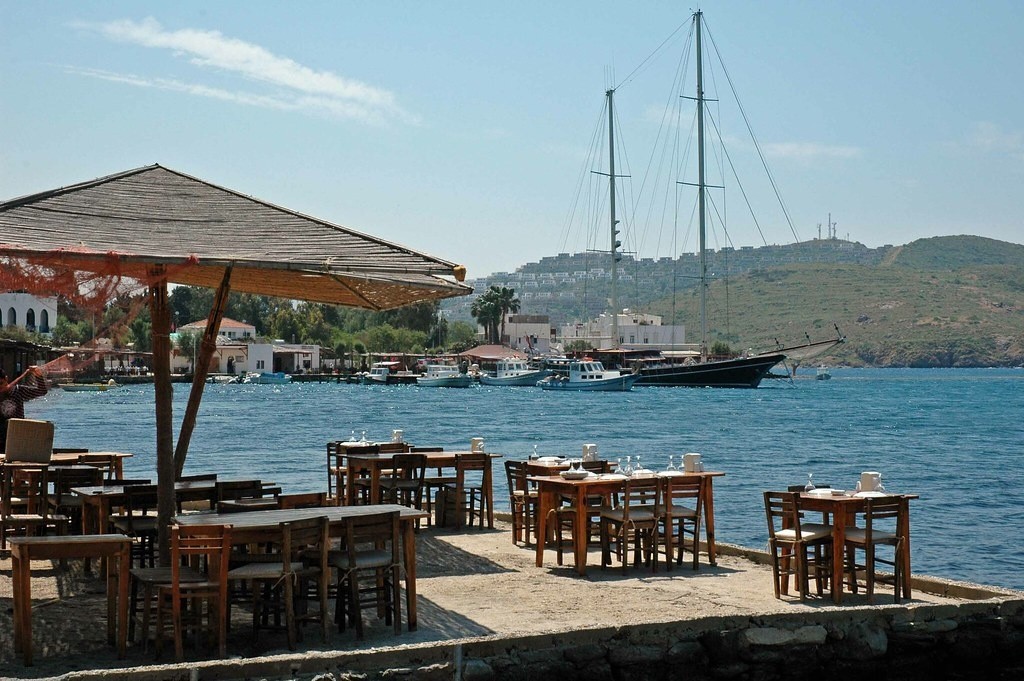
[0,164,486,564]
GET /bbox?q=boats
[416,365,471,387]
[815,362,831,380]
[361,367,389,384]
[206,372,292,384]
[479,370,553,386]
[57,380,123,392]
[536,357,643,391]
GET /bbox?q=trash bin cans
[435,490,466,527]
[173,366,189,374]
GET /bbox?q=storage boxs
[5,418,54,464]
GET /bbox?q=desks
[336,451,503,534]
[0,451,133,481]
[525,472,725,577]
[781,489,919,604]
[170,504,431,631]
[69,481,276,580]
[6,535,136,667]
[516,462,618,547]
[339,437,415,502]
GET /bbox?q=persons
[0,366,48,454]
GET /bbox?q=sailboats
[528,9,847,389]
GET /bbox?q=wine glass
[679,454,686,470]
[667,454,675,471]
[569,458,584,471]
[615,455,643,476]
[349,431,367,447]
[531,444,539,463]
[804,472,815,494]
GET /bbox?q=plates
[854,491,886,498]
[831,490,845,495]
[807,488,835,495]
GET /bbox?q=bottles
[856,481,862,493]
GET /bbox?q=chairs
[763,484,905,604]
[341,510,401,638]
[504,455,705,573]
[0,441,490,661]
